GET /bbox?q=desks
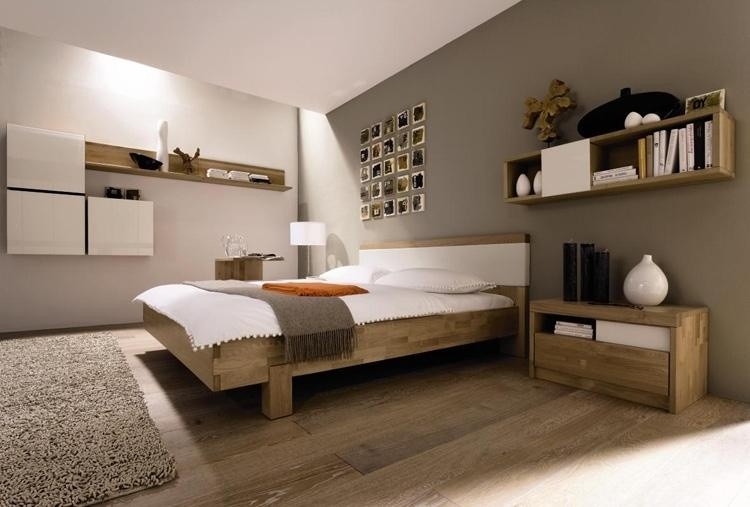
[215,255,268,281]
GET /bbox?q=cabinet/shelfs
[6,123,86,194]
[86,138,293,192]
[528,295,711,415]
[87,196,154,257]
[6,190,86,257]
[502,105,737,205]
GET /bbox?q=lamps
[290,220,327,278]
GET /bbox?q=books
[638,138,646,178]
[556,320,593,329]
[686,123,695,172]
[646,135,653,178]
[665,129,679,175]
[554,329,593,340]
[592,168,638,181]
[593,174,639,185]
[705,120,713,168]
[593,165,634,176]
[679,127,687,173]
[658,130,668,175]
[554,325,593,335]
[695,125,705,169]
[653,132,660,176]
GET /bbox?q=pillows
[376,266,497,294]
[317,263,388,287]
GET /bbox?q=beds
[129,230,530,420]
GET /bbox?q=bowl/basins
[128,152,163,169]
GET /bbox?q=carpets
[0,330,181,507]
